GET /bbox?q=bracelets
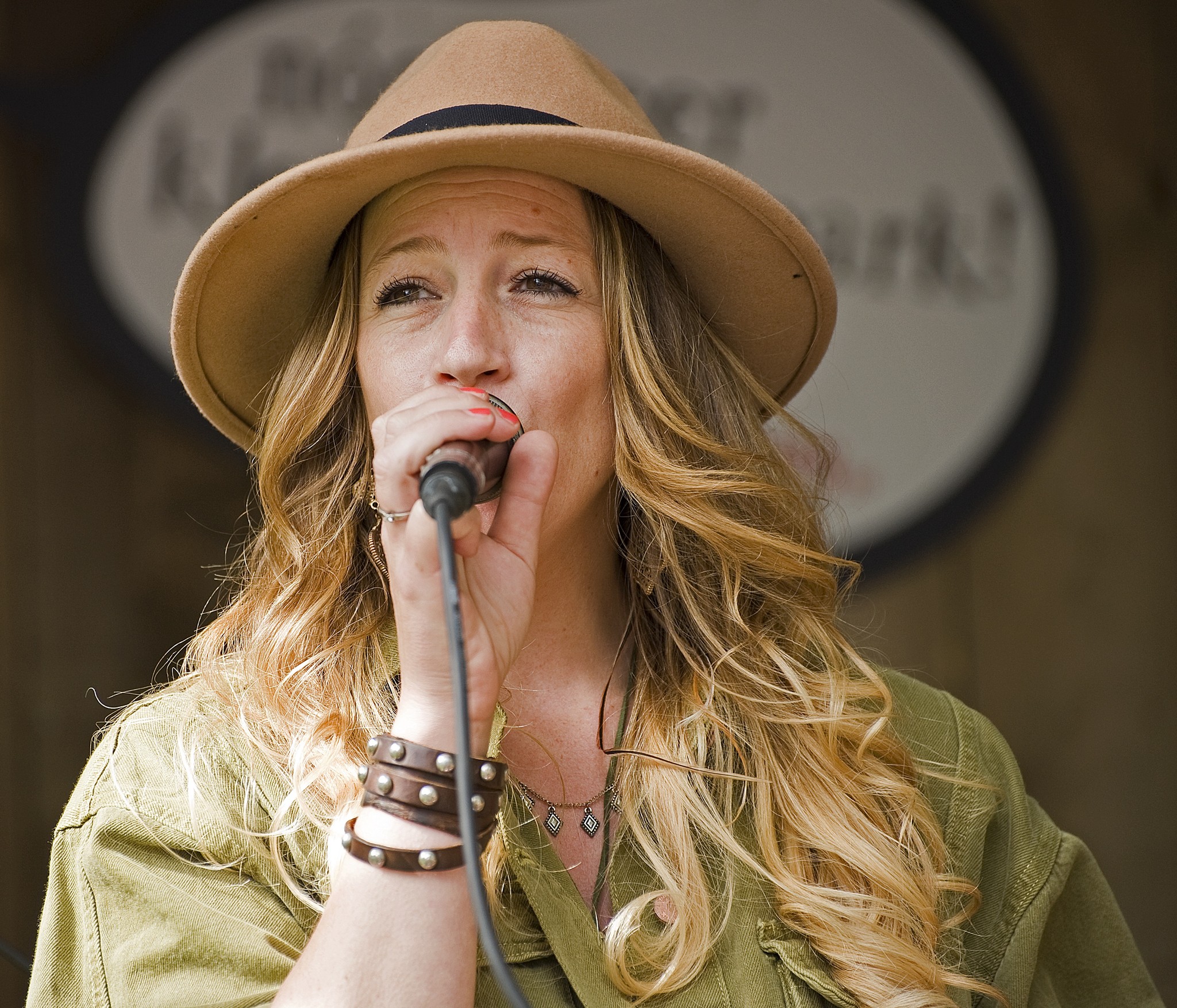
[343,735,510,875]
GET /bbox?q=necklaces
[504,762,623,839]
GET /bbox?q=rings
[376,506,409,522]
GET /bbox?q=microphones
[418,392,526,521]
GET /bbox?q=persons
[25,18,1158,1006]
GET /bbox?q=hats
[171,20,836,446]
